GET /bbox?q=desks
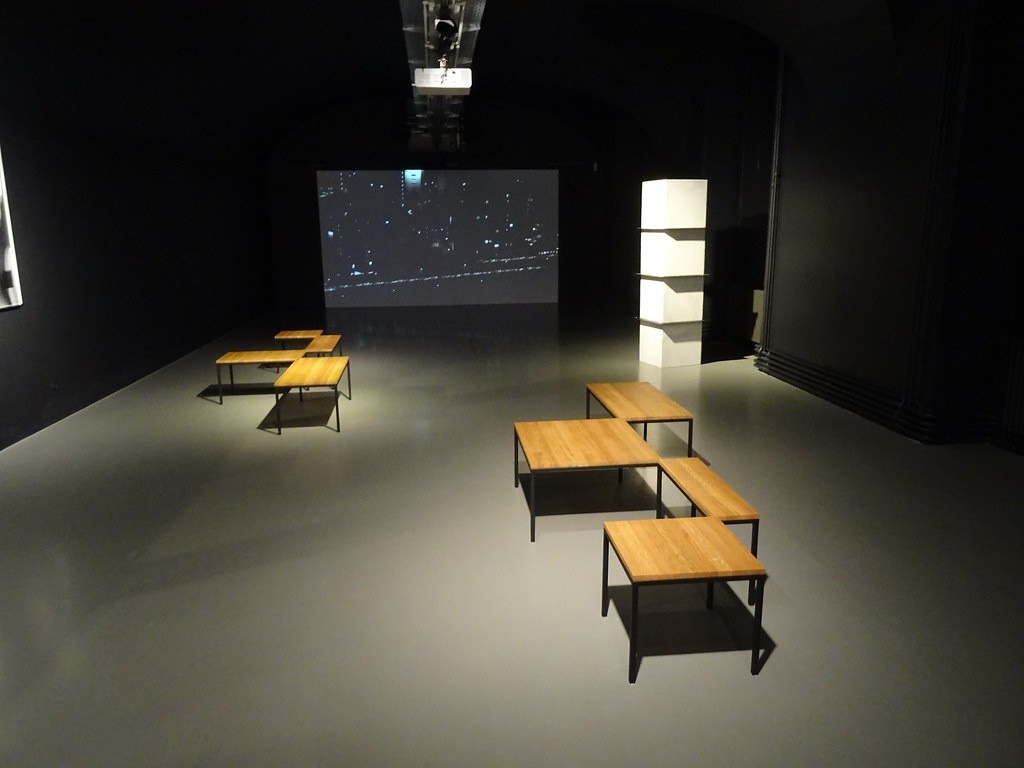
[274,356,352,435]
[602,515,769,682]
[513,419,663,542]
[307,334,343,356]
[216,348,310,405]
[586,381,695,456]
[275,329,323,350]
[656,455,760,608]
